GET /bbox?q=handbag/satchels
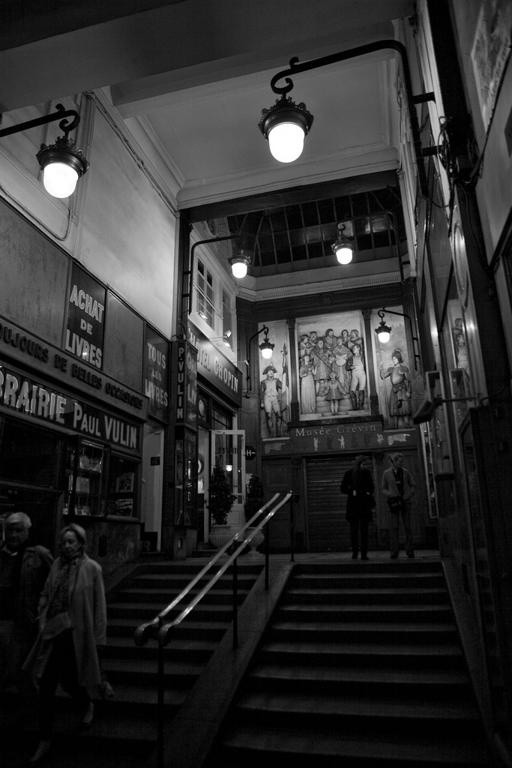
[42,612,74,640]
[389,496,406,514]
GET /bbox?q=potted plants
[240,470,268,559]
[204,462,236,560]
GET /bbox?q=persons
[299,328,367,414]
[0,512,55,698]
[259,366,283,435]
[339,454,376,560]
[380,451,417,560]
[380,351,414,426]
[23,523,116,764]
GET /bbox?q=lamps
[254,37,443,202]
[1,99,91,201]
[243,320,278,400]
[375,299,420,373]
[324,207,412,293]
[181,232,256,316]
